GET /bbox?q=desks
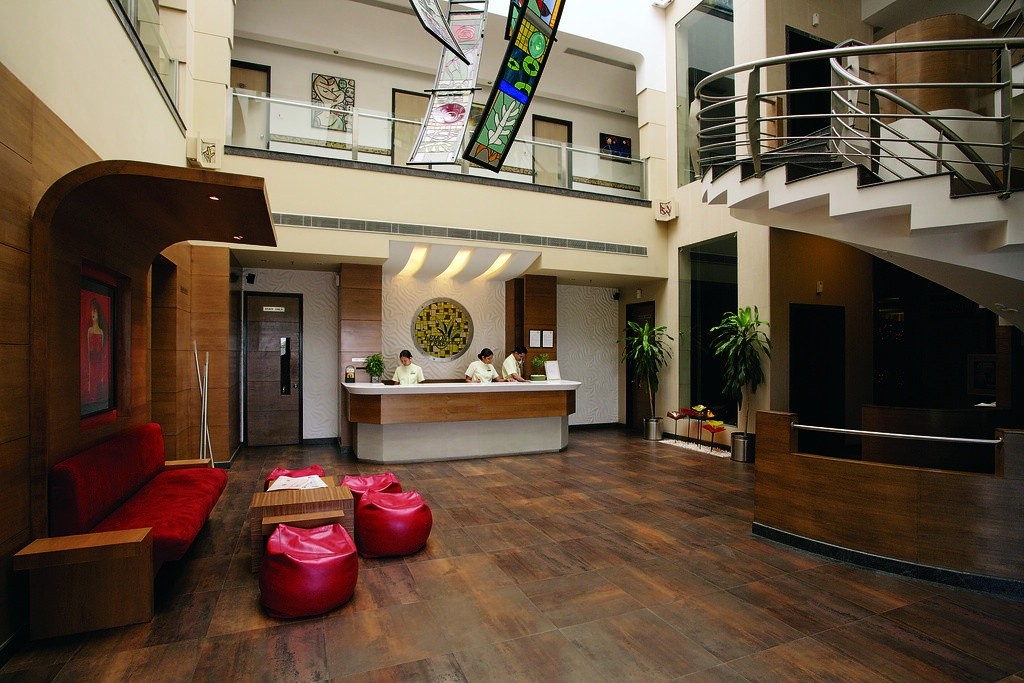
[247,476,355,576]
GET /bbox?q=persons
[465,348,515,383]
[392,350,425,385]
[502,346,530,381]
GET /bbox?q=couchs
[13,420,229,645]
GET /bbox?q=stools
[339,471,403,525]
[259,523,358,620]
[264,464,325,493]
[356,488,433,562]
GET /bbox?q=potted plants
[530,354,549,380]
[615,321,674,441]
[709,305,773,463]
[362,352,386,383]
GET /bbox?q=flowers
[681,405,715,448]
[666,411,686,443]
[702,420,726,453]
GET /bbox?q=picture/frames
[79,272,116,420]
